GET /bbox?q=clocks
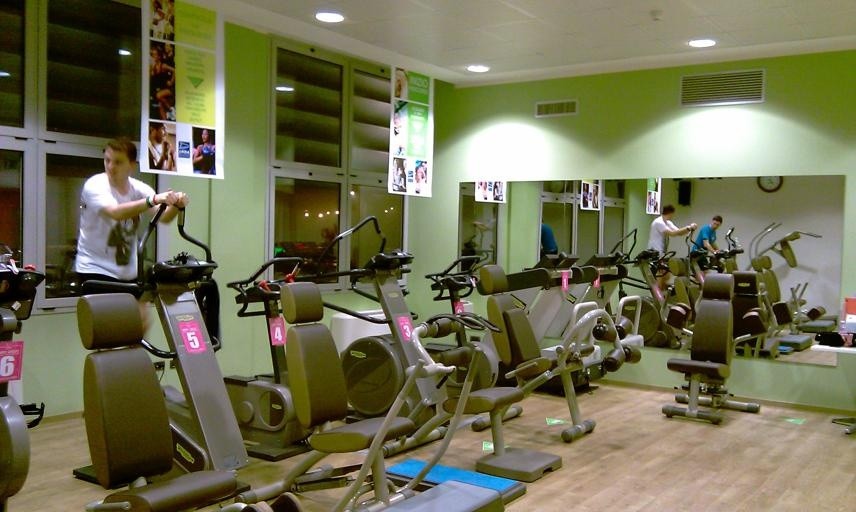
[757,176,785,193]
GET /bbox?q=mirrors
[454,175,847,368]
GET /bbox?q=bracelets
[173,203,185,211]
[146,194,156,207]
[687,226,690,231]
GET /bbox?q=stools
[445,384,566,481]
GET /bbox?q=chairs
[470,262,596,444]
[660,272,761,427]
[76,292,252,511]
[281,277,414,509]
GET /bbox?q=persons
[415,161,427,193]
[541,217,559,259]
[648,205,693,289]
[72,140,189,295]
[192,129,217,176]
[148,0,176,170]
[474,206,497,262]
[321,226,335,256]
[479,181,487,200]
[493,181,503,201]
[688,215,723,274]
[648,192,658,213]
[592,185,598,208]
[397,166,406,191]
